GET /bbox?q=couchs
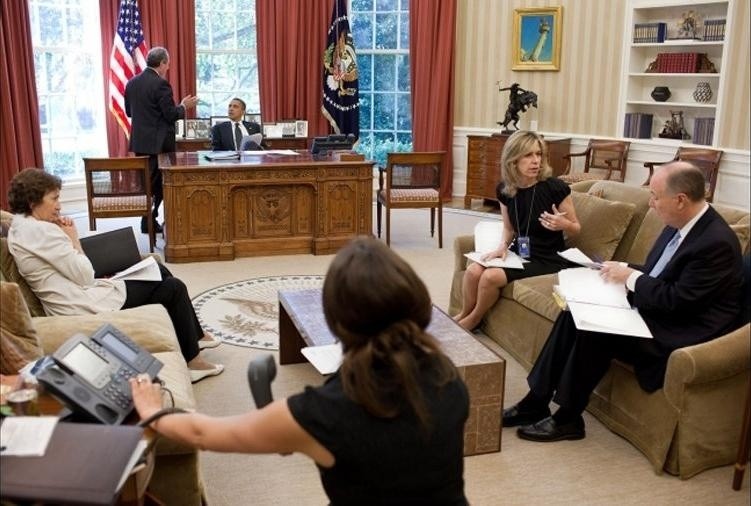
[0,209,207,505]
[448,180,751,479]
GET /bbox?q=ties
[648,231,682,278]
[234,122,242,149]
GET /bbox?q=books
[624,18,727,146]
[104,255,163,282]
[299,341,347,377]
[204,150,241,161]
[463,248,532,271]
[552,263,654,340]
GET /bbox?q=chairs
[83,156,156,253]
[642,147,723,203]
[561,139,631,183]
[376,151,448,248]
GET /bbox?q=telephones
[248,353,294,457]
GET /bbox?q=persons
[129,236,471,506]
[451,129,583,331]
[211,96,270,151]
[0,416,156,506]
[6,168,226,385]
[500,162,751,442]
[499,82,530,113]
[123,45,202,234]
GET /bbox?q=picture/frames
[511,6,562,71]
[175,114,308,140]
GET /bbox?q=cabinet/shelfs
[175,140,310,152]
[464,134,571,211]
[616,0,737,148]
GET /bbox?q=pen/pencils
[204,155,211,161]
[592,253,605,263]
[556,211,568,216]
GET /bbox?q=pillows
[562,190,637,264]
[587,180,651,261]
[626,205,751,266]
[0,237,46,317]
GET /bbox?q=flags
[320,0,360,147]
[108,0,150,142]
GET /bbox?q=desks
[158,152,376,264]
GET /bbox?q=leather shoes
[189,364,225,384]
[142,226,163,233]
[198,336,221,351]
[517,414,586,443]
[502,404,552,428]
